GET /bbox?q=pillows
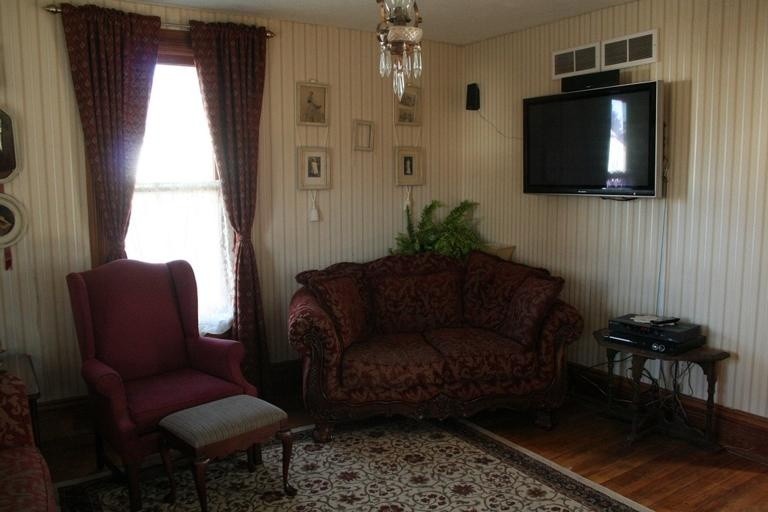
[364,270,466,332]
[293,268,377,350]
[463,252,568,351]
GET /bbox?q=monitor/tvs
[522,82,667,198]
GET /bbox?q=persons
[309,157,321,177]
[303,91,322,123]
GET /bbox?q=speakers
[466,82,479,109]
[560,69,621,90]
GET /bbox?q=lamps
[369,0,428,102]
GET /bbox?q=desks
[592,327,730,448]
[1,352,42,449]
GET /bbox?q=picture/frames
[390,83,423,127]
[0,106,21,184]
[392,145,427,187]
[295,80,330,128]
[350,119,375,152]
[0,192,28,249]
[295,144,333,192]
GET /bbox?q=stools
[148,390,300,512]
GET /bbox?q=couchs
[282,244,586,448]
[0,367,60,511]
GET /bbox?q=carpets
[48,407,664,512]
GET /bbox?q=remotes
[652,315,681,325]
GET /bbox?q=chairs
[65,256,266,509]
